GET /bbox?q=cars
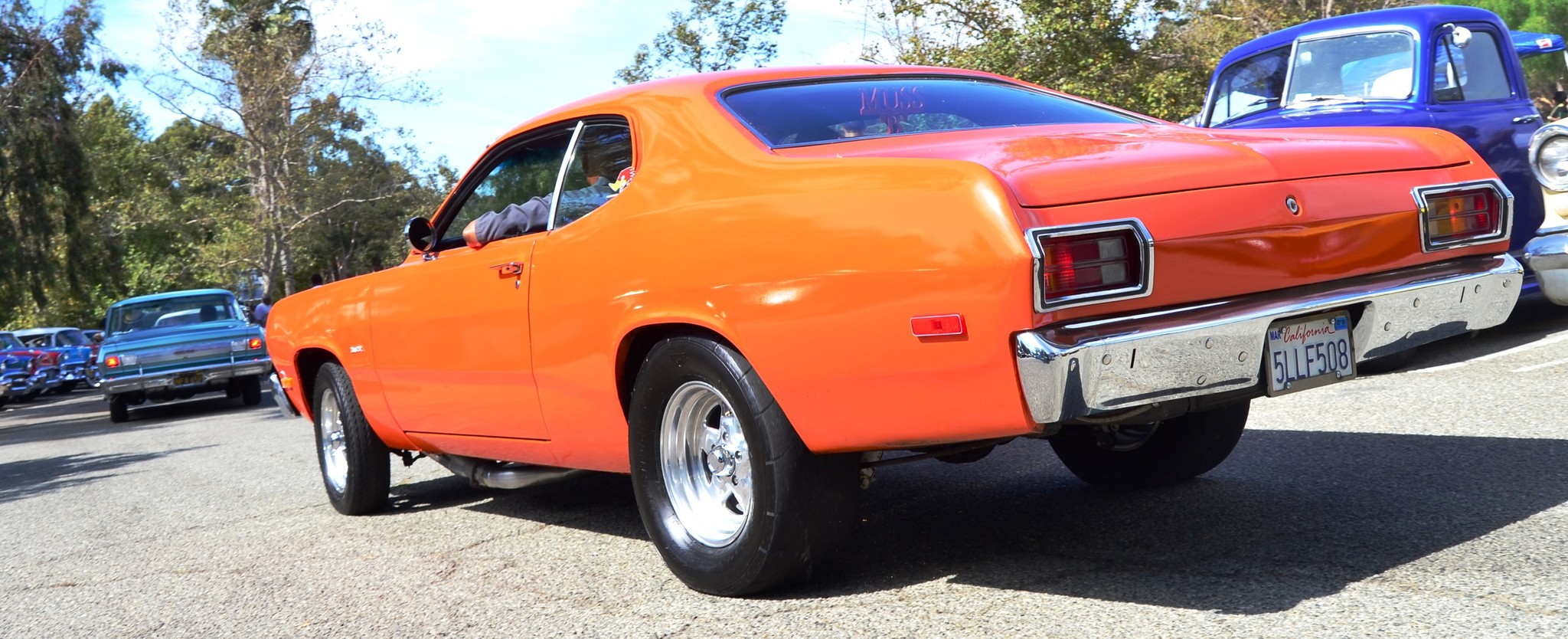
[260,64,1523,593]
[0,288,274,422]
[1176,1,1568,255]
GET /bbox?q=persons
[254,293,272,327]
[200,304,218,322]
[93,318,115,341]
[311,274,323,287]
[463,140,632,247]
[123,310,146,329]
